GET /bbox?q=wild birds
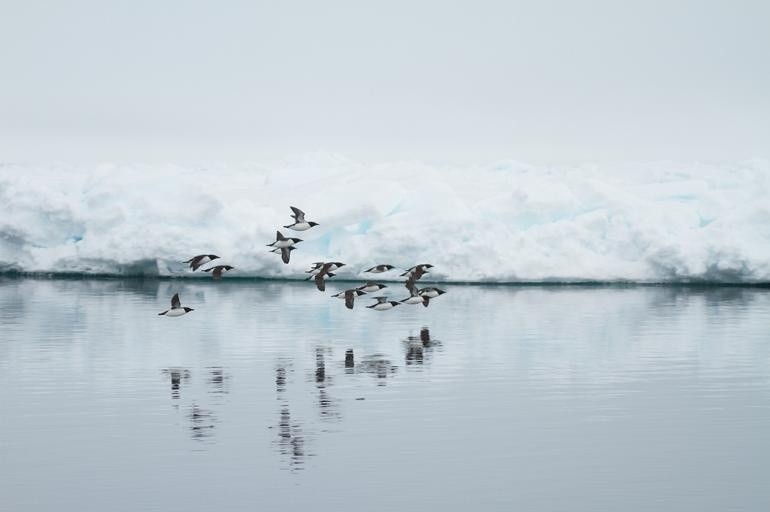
[303,258,447,310]
[181,253,233,280]
[267,205,317,262]
[156,292,194,319]
